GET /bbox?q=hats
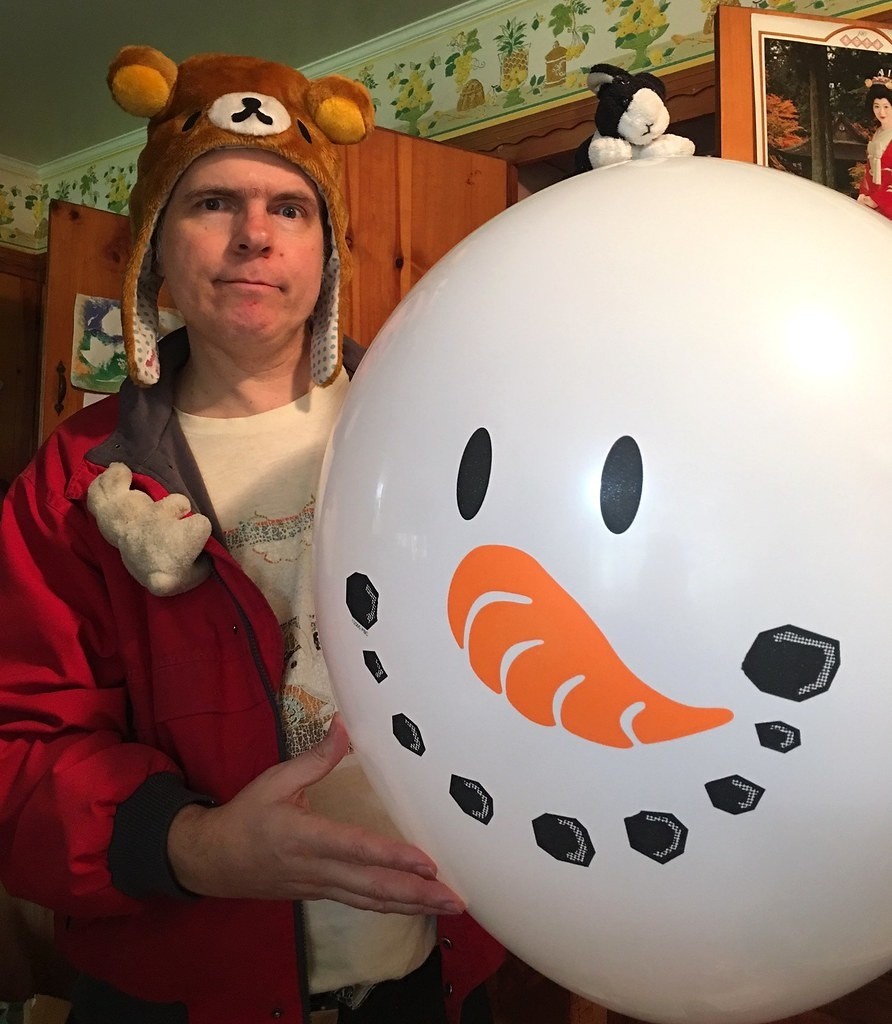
[106,43,373,391]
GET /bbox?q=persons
[856,76,892,222]
[0,45,504,1024]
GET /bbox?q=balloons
[314,158,892,1023]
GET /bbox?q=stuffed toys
[574,64,696,174]
[86,463,213,597]
